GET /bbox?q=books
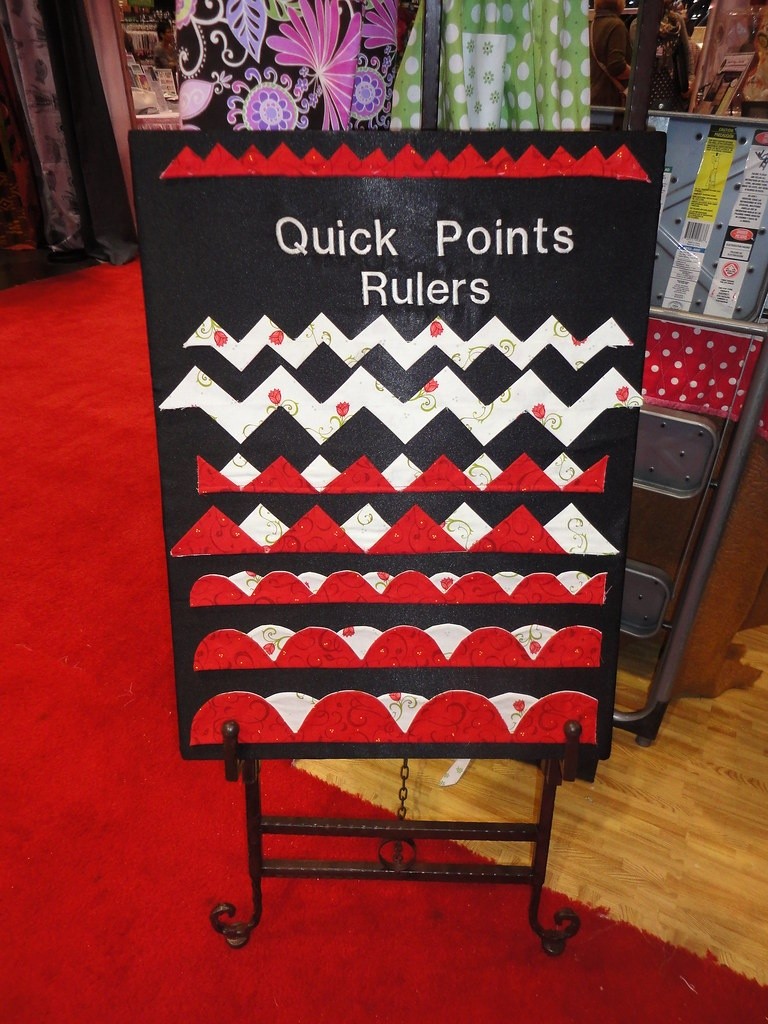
[126,53,158,91]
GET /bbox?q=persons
[683,0,712,27]
[587,0,632,111]
[628,0,690,110]
[153,20,178,89]
